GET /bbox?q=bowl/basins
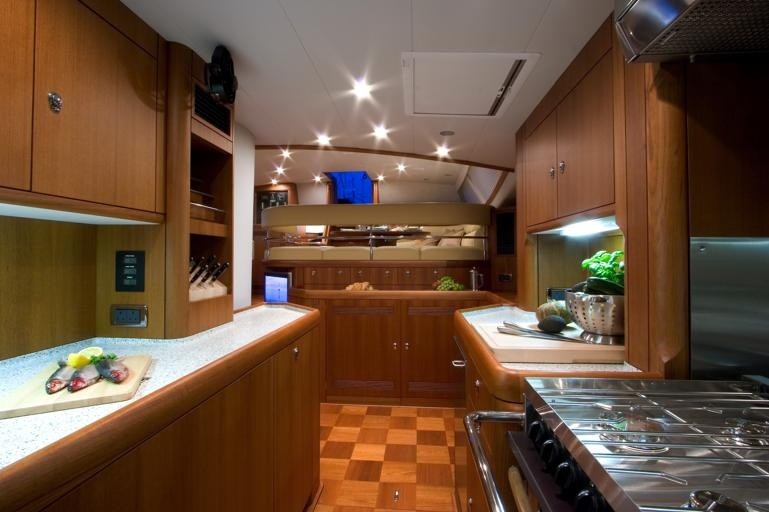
[565,290,624,345]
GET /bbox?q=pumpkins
[536,300,576,324]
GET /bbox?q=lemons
[68,346,103,368]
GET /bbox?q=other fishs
[96,359,129,383]
[69,364,101,393]
[46,361,78,394]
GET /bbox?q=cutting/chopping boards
[0,354,154,420]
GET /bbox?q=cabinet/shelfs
[276,304,323,512]
[30,2,165,222]
[0,2,38,192]
[0,359,276,512]
[524,47,615,229]
[99,40,236,340]
[253,182,298,230]
[324,293,486,407]
[261,206,497,291]
[464,348,524,512]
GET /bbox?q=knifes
[198,263,220,286]
[190,256,205,276]
[212,262,230,283]
[190,264,209,287]
